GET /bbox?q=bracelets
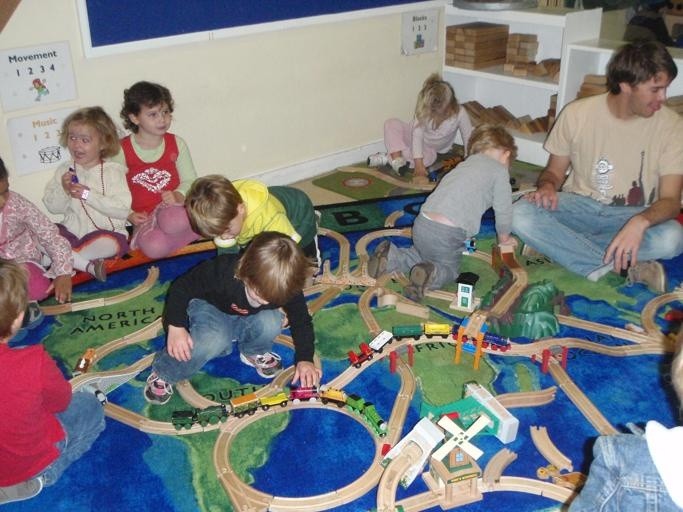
[81,189,90,201]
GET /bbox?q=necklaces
[73,162,115,234]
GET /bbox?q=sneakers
[627,260,666,294]
[521,244,544,256]
[408,262,433,298]
[0,478,44,504]
[368,240,391,278]
[367,152,410,177]
[21,301,43,329]
[144,370,173,406]
[239,351,283,379]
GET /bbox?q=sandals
[86,258,109,283]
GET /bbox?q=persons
[368,126,519,302]
[106,80,202,264]
[141,231,324,405]
[363,73,475,180]
[39,104,134,284]
[183,174,323,256]
[0,261,106,503]
[0,157,74,308]
[562,306,682,512]
[621,0,682,49]
[509,39,682,295]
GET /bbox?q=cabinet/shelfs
[441,5,603,172]
[560,39,683,118]
